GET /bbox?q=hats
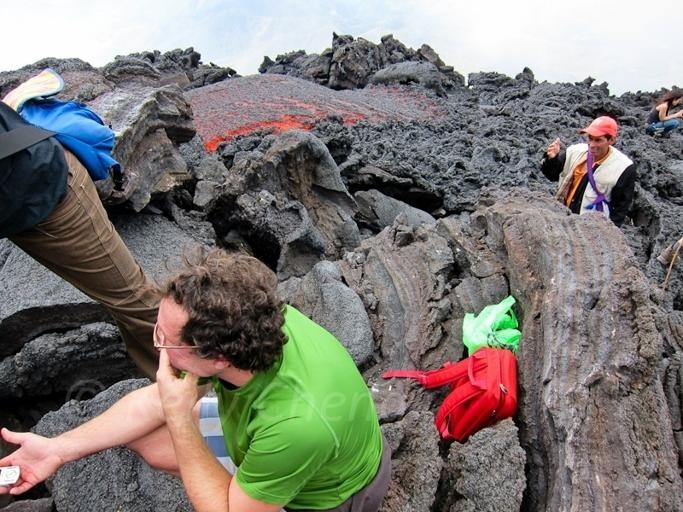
[580,116,618,139]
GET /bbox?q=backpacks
[420,346,519,443]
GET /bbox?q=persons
[0,243,391,512]
[0,100,162,383]
[539,116,635,228]
[645,91,683,136]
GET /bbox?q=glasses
[152,323,202,352]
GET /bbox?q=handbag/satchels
[16,92,116,181]
